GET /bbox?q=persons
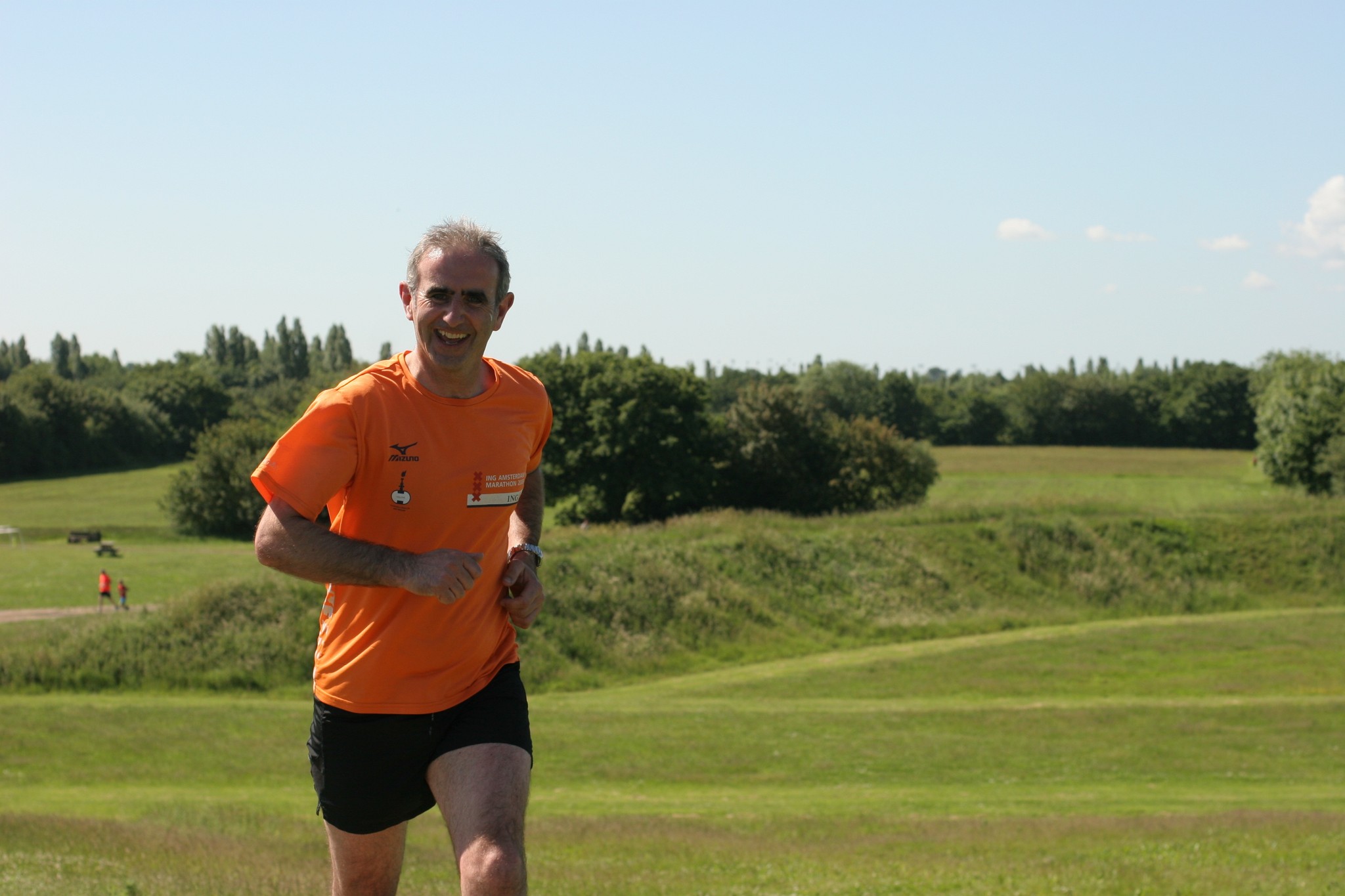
[97,568,118,613]
[247,219,553,895]
[117,579,130,611]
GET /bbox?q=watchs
[507,542,543,568]
